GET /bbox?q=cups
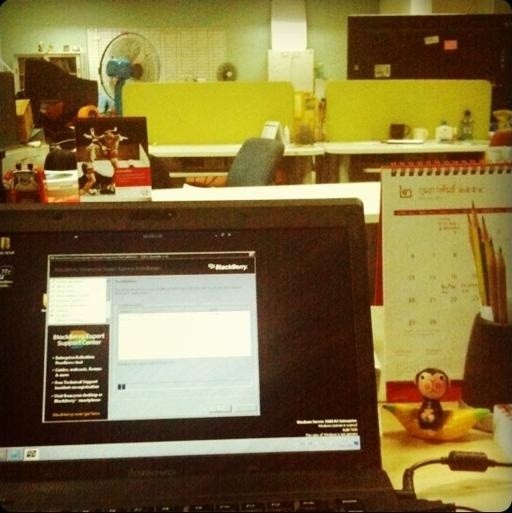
[459,311,512,412]
[389,122,411,139]
[412,128,429,140]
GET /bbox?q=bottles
[298,97,323,142]
[458,109,475,140]
[435,121,454,140]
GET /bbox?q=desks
[147,141,325,189]
[315,141,489,183]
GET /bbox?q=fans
[99,31,161,117]
[215,63,237,80]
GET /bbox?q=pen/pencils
[467,200,508,324]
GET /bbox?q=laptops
[0,198,456,513]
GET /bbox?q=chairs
[225,138,285,186]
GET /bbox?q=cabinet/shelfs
[12,52,83,96]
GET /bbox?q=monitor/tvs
[122,80,295,145]
[326,79,493,142]
[23,58,98,135]
